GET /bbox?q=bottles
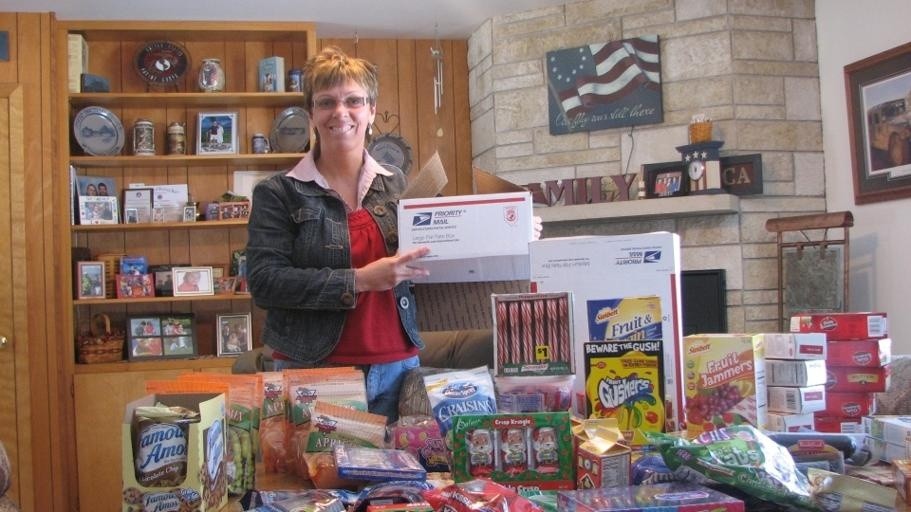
[132,119,155,156]
[166,121,186,154]
[251,133,266,153]
[289,68,303,92]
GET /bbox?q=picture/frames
[842,40,911,206]
[719,153,764,196]
[640,160,685,198]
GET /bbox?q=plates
[269,106,310,153]
[72,105,126,157]
[367,132,413,176]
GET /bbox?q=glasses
[311,97,371,110]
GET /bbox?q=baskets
[77,313,125,364]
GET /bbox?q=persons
[178,271,201,291]
[86,183,110,196]
[246,44,543,442]
[134,319,187,355]
[206,121,224,147]
[154,209,162,221]
[655,176,678,196]
[222,322,246,352]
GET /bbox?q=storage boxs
[394,147,536,284]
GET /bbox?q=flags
[546,35,660,120]
